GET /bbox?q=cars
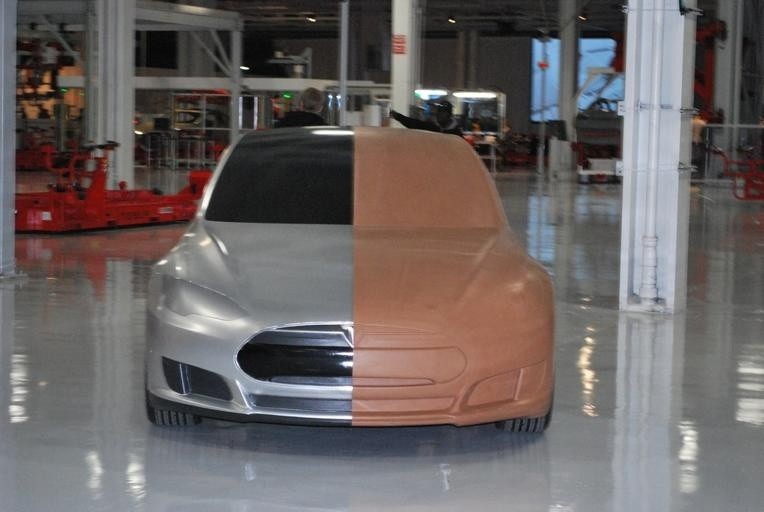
[142,123,557,440]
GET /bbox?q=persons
[471,117,481,132]
[272,87,331,129]
[386,100,465,139]
